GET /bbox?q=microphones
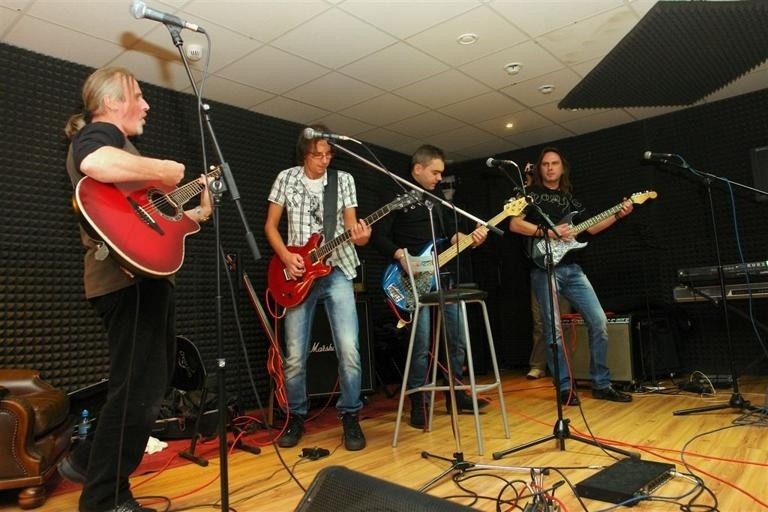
[641,151,675,164]
[484,155,514,171]
[128,1,198,37]
[303,126,343,147]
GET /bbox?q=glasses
[308,150,337,160]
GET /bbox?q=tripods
[416,256,550,492]
[670,234,768,418]
[492,291,651,463]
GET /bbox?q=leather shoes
[560,388,581,407]
[444,394,491,415]
[409,400,429,430]
[591,386,633,403]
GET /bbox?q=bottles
[78,410,93,443]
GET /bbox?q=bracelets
[532,225,543,239]
[194,205,212,225]
[613,211,622,220]
[400,253,405,259]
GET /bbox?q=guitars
[267,190,422,308]
[383,195,534,312]
[522,190,658,270]
[73,166,222,276]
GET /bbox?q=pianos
[673,260,768,303]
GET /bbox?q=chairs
[1,367,80,510]
[391,245,510,459]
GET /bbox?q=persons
[509,146,639,408]
[518,158,573,383]
[262,123,375,451]
[57,65,225,512]
[366,143,489,428]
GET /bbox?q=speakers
[292,464,485,512]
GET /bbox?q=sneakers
[108,494,159,512]
[527,368,547,380]
[278,414,305,448]
[341,412,366,452]
[56,453,87,484]
